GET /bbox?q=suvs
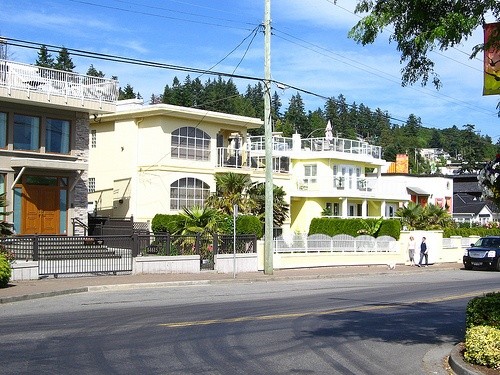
[462,236,500,271]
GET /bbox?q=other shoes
[417,264,422,267]
[425,264,428,268]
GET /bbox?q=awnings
[407,186,430,196]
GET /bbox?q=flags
[483,22,500,96]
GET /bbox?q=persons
[408,236,416,267]
[417,236,428,267]
